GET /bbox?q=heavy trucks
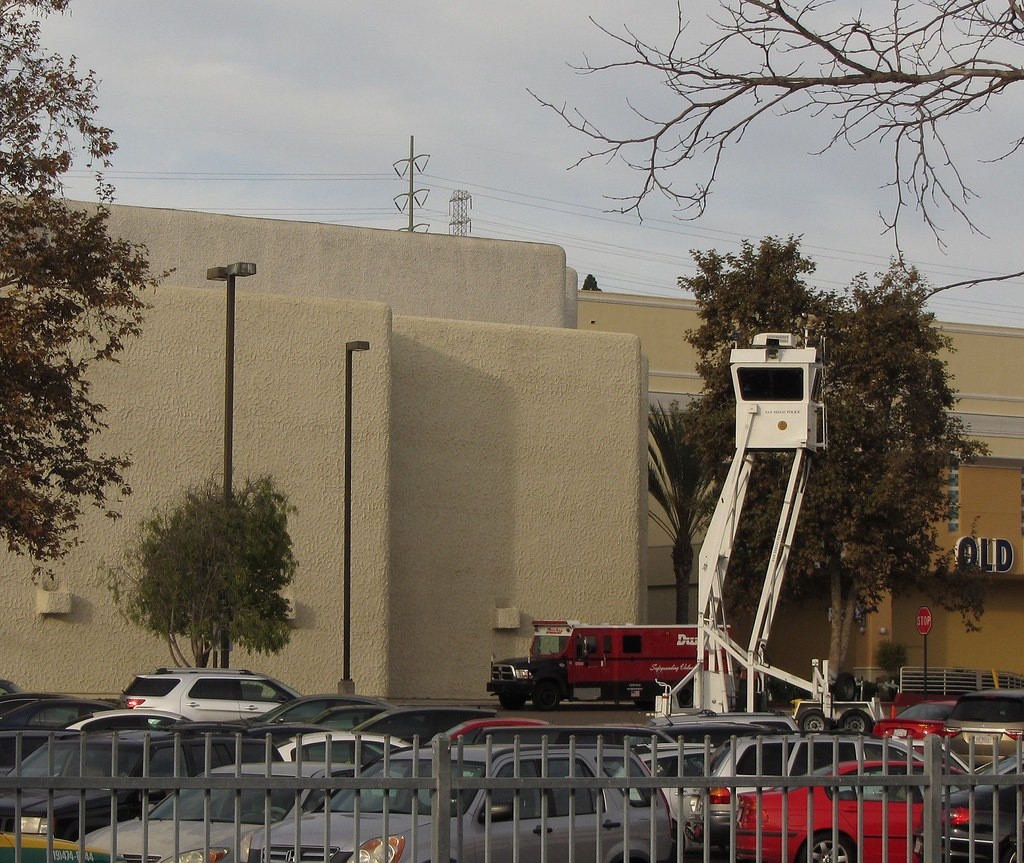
[485,619,735,710]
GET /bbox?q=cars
[945,688,1024,765]
[614,740,725,853]
[873,698,957,757]
[74,759,438,862]
[0,678,803,769]
[728,758,969,863]
[912,754,1024,863]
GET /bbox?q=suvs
[0,718,340,844]
[236,742,675,863]
[688,729,978,846]
[118,664,311,727]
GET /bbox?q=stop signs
[916,607,933,635]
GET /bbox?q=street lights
[205,261,258,667]
[337,338,374,697]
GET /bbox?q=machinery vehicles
[656,332,875,734]
